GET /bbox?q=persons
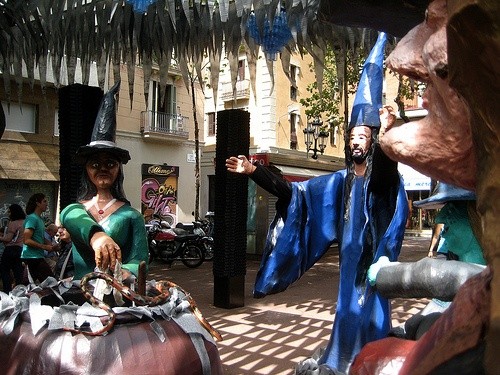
[19,193,58,287]
[411,181,489,265]
[0,202,26,292]
[59,136,151,279]
[223,32,408,375]
[54,224,74,281]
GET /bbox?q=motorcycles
[145,211,215,269]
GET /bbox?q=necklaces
[92,194,117,214]
